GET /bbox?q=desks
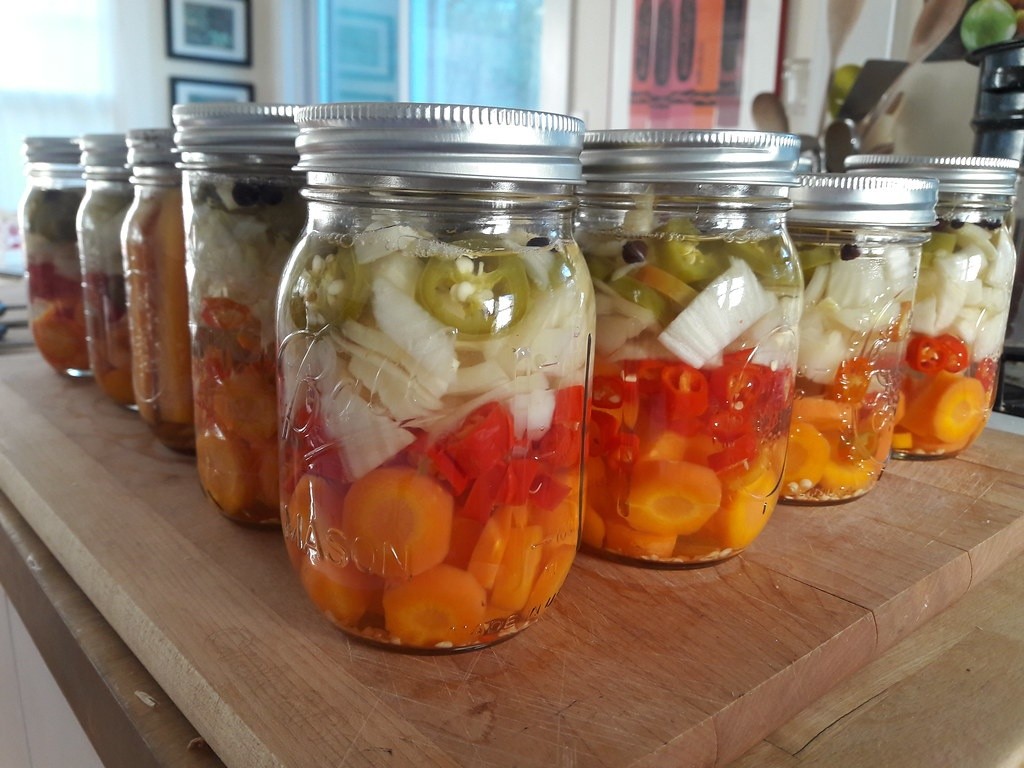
[0,275,1019,767]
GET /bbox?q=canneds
[25,102,1017,655]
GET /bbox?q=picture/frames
[170,77,255,127]
[166,0,252,67]
